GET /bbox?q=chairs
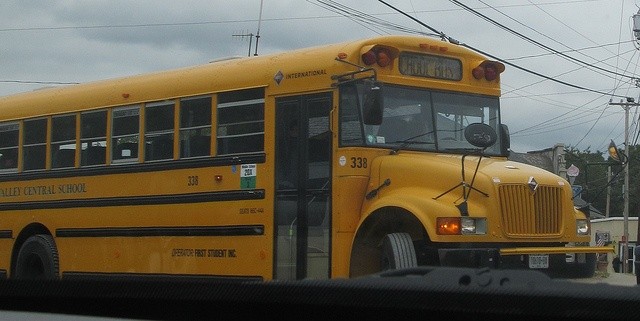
[82,146,106,166]
[55,149,75,168]
[223,135,256,154]
[114,143,137,158]
[148,141,171,160]
[190,135,211,156]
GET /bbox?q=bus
[0,35,613,284]
